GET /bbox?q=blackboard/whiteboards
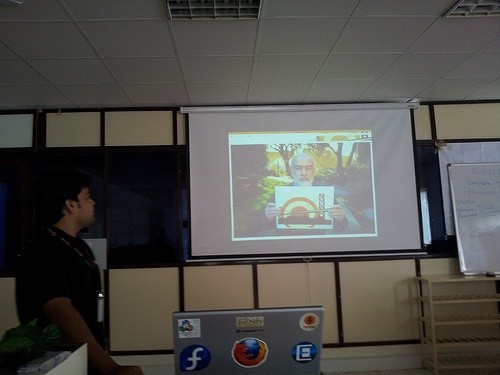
[449,162,500,275]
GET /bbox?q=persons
[257,153,348,233]
[15,166,146,375]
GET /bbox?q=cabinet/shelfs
[415,274,500,375]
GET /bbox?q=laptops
[172,305,325,375]
